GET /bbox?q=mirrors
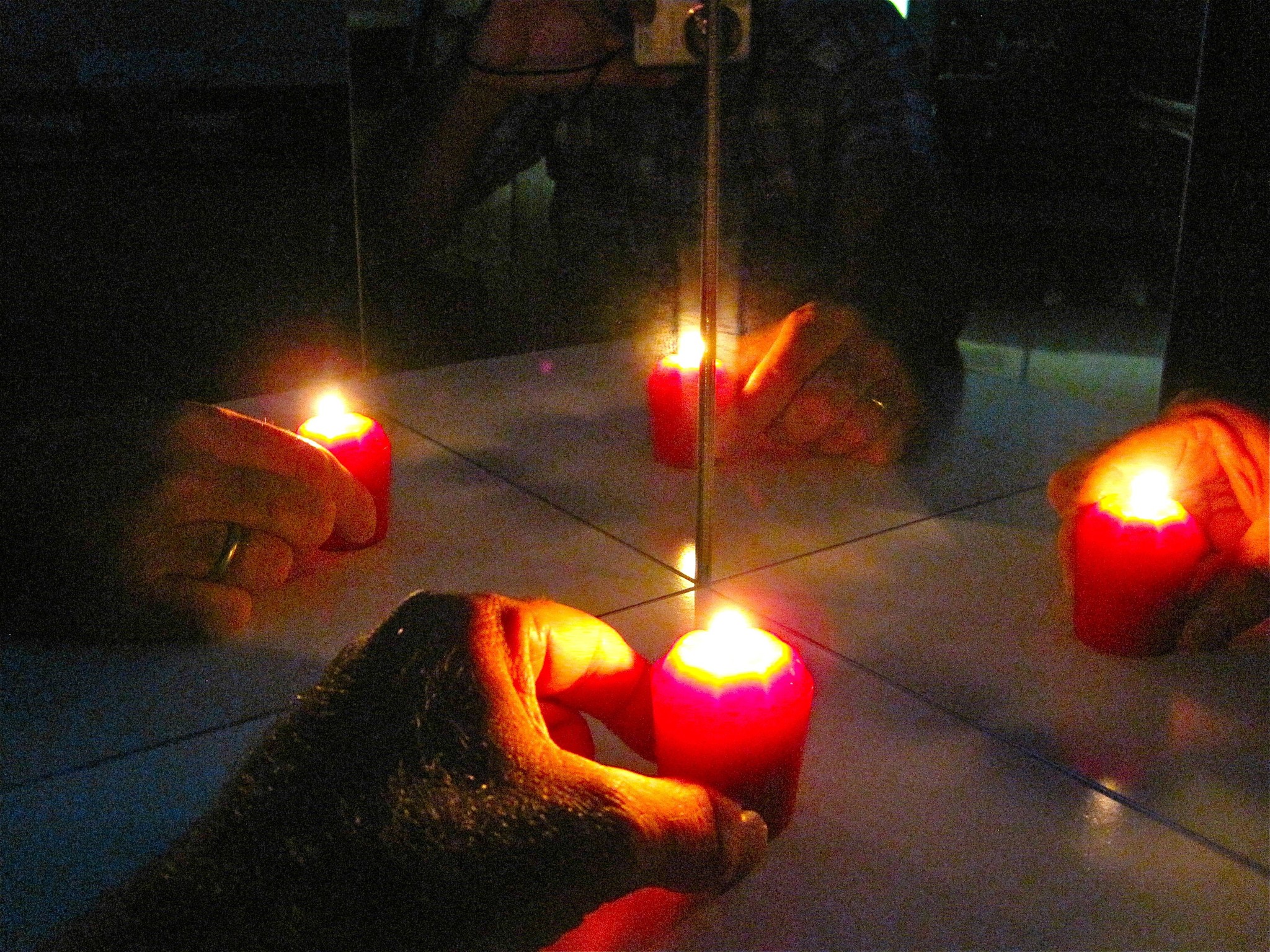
[0,0,1270,870]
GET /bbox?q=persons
[1057,3,1270,649]
[3,0,974,649]
[21,589,769,951]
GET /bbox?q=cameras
[633,0,750,65]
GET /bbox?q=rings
[863,389,895,419]
[208,520,244,582]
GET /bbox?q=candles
[1075,467,1204,656]
[646,326,730,468]
[647,600,812,840]
[297,387,391,551]
